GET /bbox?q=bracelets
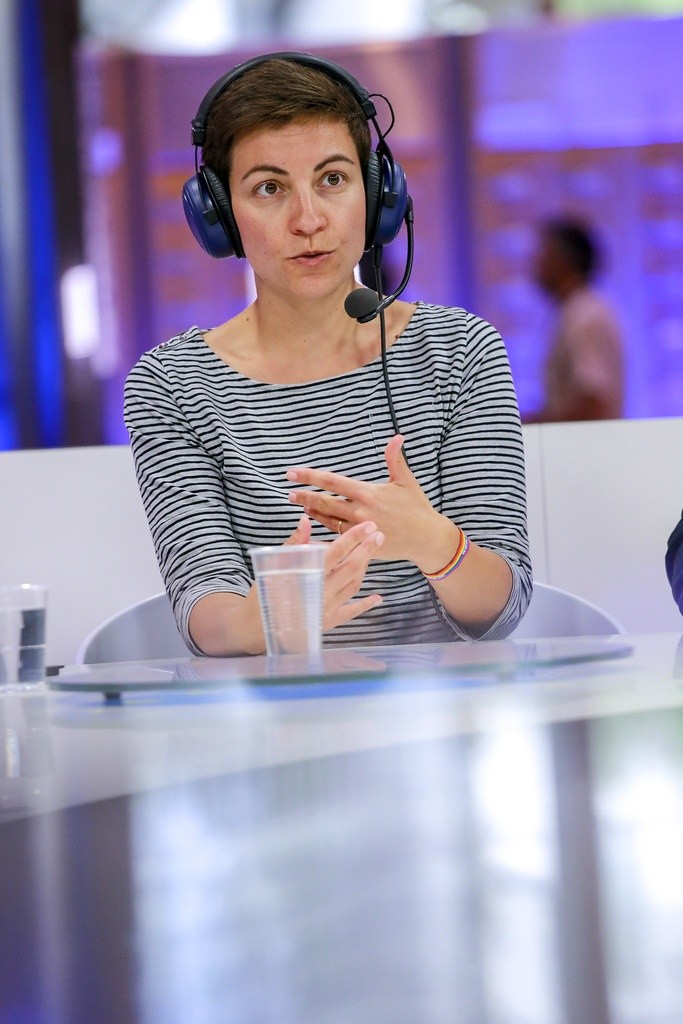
[421,527,469,580]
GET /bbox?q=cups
[0,585,49,691]
[247,544,330,658]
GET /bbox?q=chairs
[77,581,629,664]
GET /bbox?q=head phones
[180,51,413,259]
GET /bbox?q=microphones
[343,222,415,322]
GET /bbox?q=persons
[124,52,532,657]
[527,216,622,421]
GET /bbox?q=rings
[338,521,341,533]
